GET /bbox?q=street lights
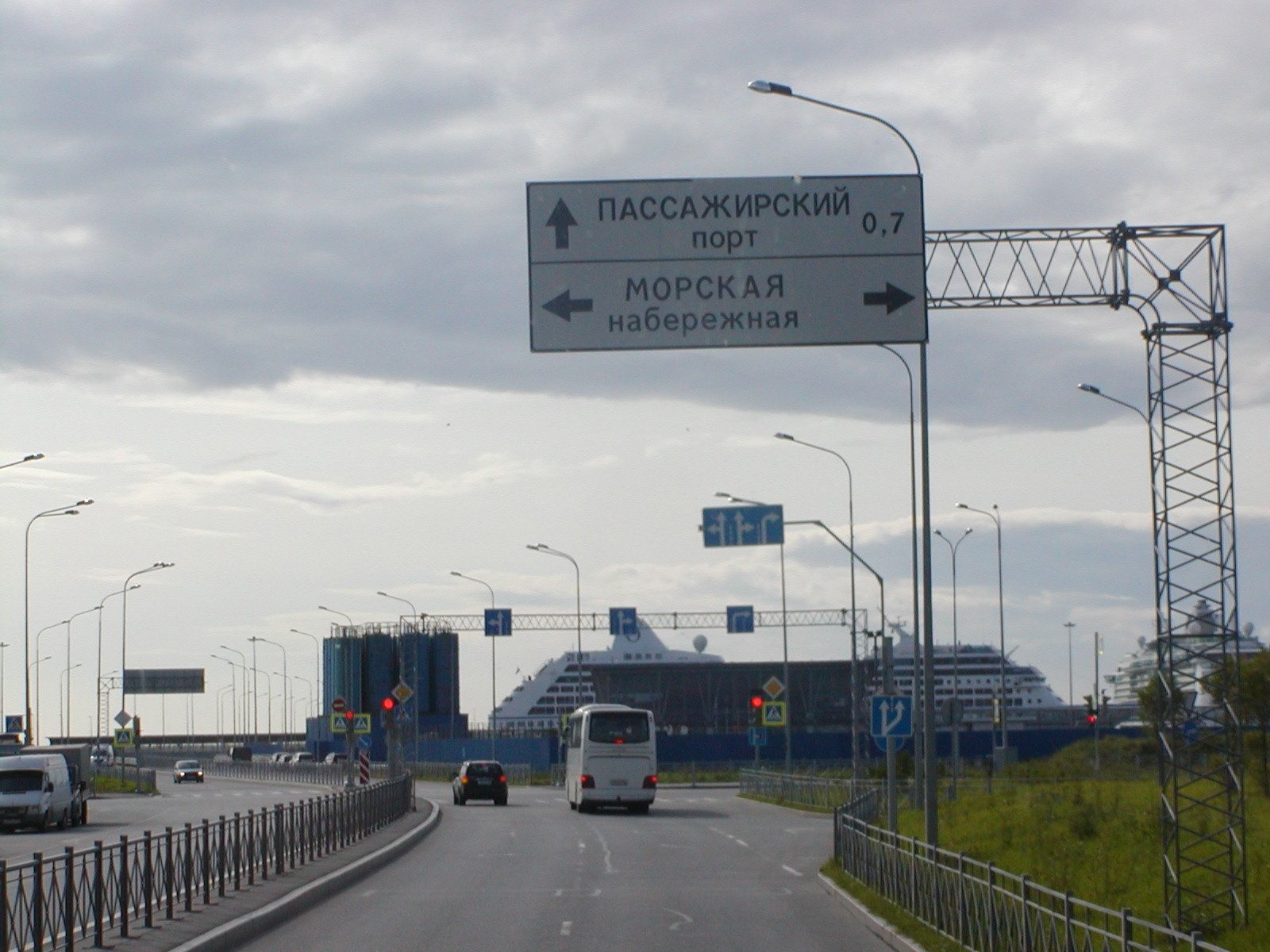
[526,542,583,708]
[774,432,859,803]
[933,527,975,780]
[375,589,419,775]
[448,570,495,761]
[747,78,938,868]
[953,502,1009,748]
[210,606,356,762]
[25,498,96,747]
[712,490,792,777]
[0,560,175,794]
[1063,621,1075,727]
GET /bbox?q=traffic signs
[524,172,926,355]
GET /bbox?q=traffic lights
[1088,709,1098,727]
[750,687,764,716]
[345,711,354,733]
[383,698,395,729]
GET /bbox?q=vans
[90,744,114,768]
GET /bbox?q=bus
[566,703,657,816]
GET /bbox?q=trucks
[0,753,92,835]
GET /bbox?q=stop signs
[332,699,345,712]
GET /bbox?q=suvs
[452,760,508,806]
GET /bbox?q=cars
[174,760,205,784]
[228,745,348,770]
